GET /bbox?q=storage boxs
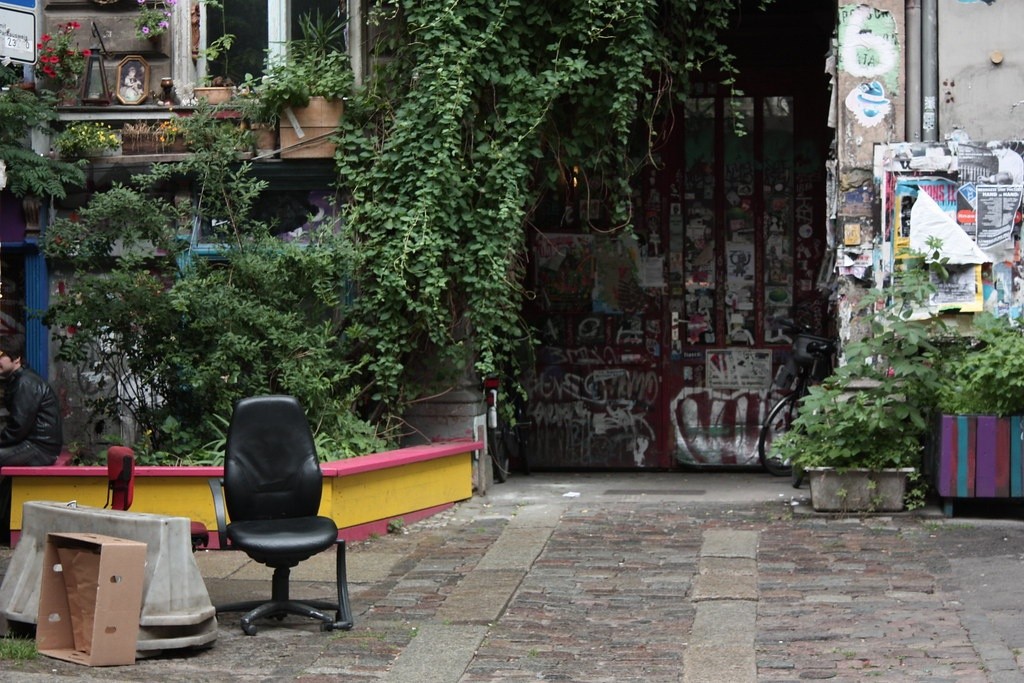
[35,532,147,667]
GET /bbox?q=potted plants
[763,233,961,510]
[121,118,167,154]
[923,306,1024,518]
[194,75,237,105]
[235,6,361,161]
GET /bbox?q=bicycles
[757,319,837,489]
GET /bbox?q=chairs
[103,446,207,554]
[208,393,354,632]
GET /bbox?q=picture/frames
[116,54,151,105]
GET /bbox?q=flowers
[159,115,197,153]
[35,20,92,89]
[51,119,124,159]
[133,0,177,40]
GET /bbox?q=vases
[164,132,190,153]
[146,34,163,51]
[57,81,79,106]
[78,151,103,158]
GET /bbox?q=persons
[120,67,144,101]
